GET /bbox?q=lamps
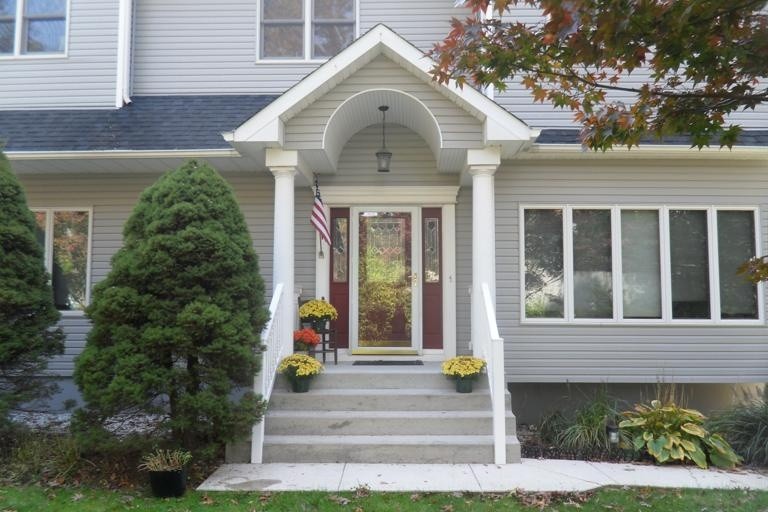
[375,105,393,173]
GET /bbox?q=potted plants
[142,448,192,498]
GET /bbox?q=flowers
[299,299,338,321]
[440,355,487,377]
[276,354,324,377]
[294,328,321,347]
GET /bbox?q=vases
[456,375,473,393]
[296,342,309,350]
[311,316,326,332]
[291,372,311,392]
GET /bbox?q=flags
[310,196,332,246]
